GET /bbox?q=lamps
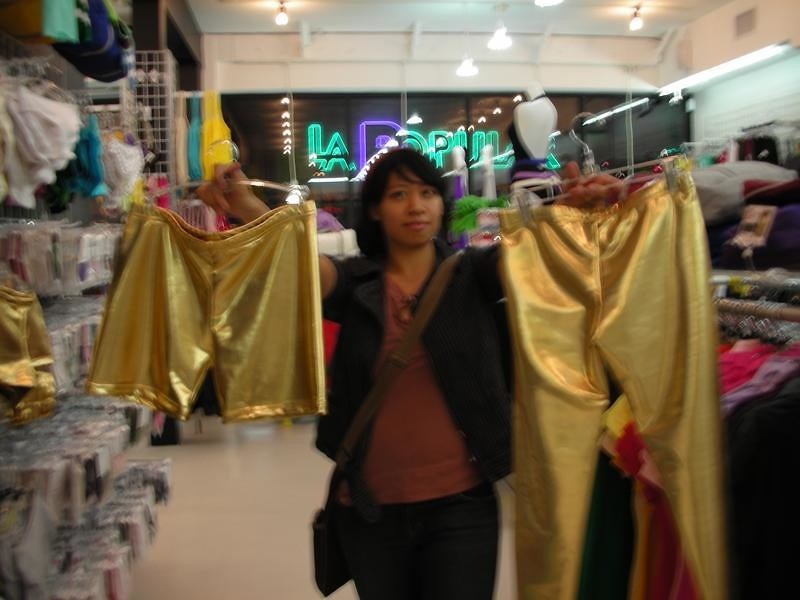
[627,6,643,30]
[581,96,649,126]
[275,0,288,26]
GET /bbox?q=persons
[198,146,625,600]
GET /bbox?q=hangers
[149,140,308,206]
[714,292,800,346]
[510,109,687,210]
[0,56,139,144]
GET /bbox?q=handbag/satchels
[312,508,354,597]
